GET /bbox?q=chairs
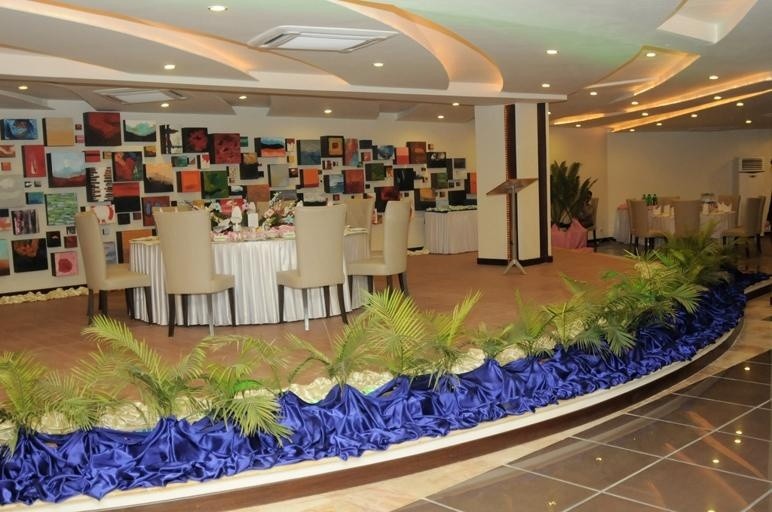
[586,198,600,252]
[276,204,348,330]
[628,193,767,258]
[74,210,152,326]
[152,209,236,337]
[333,198,376,256]
[346,200,410,313]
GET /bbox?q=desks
[425,209,478,255]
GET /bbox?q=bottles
[642,193,657,206]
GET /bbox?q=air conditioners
[738,156,765,236]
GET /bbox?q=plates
[132,238,151,241]
[347,228,366,231]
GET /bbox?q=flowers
[182,191,304,234]
